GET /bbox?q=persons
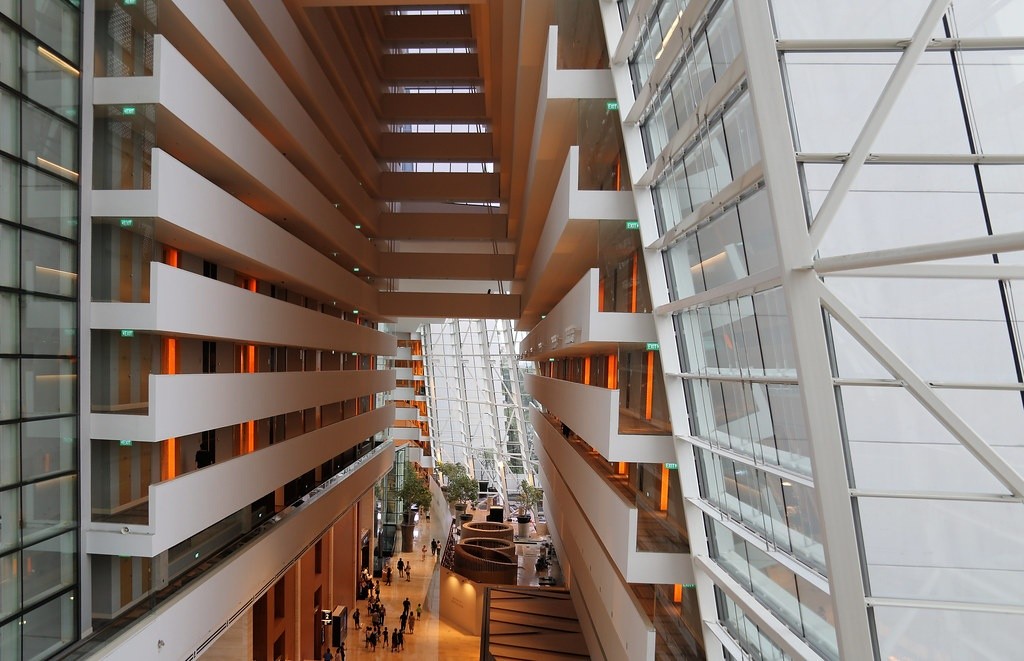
[408,611,416,634]
[361,566,375,598]
[431,538,437,556]
[400,611,407,630]
[397,629,406,653]
[425,508,431,523]
[352,607,363,630]
[382,626,389,649]
[375,580,380,602]
[335,648,342,661]
[390,628,397,653]
[340,642,347,661]
[435,540,442,555]
[323,648,334,661]
[367,595,386,627]
[365,626,382,652]
[404,561,411,582]
[422,545,427,561]
[397,558,404,578]
[386,567,392,587]
[402,597,411,617]
[416,604,422,621]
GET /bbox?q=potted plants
[400,464,424,523]
[457,475,478,521]
[394,480,433,552]
[517,480,543,523]
[443,461,468,510]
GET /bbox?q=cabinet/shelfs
[332,604,348,647]
